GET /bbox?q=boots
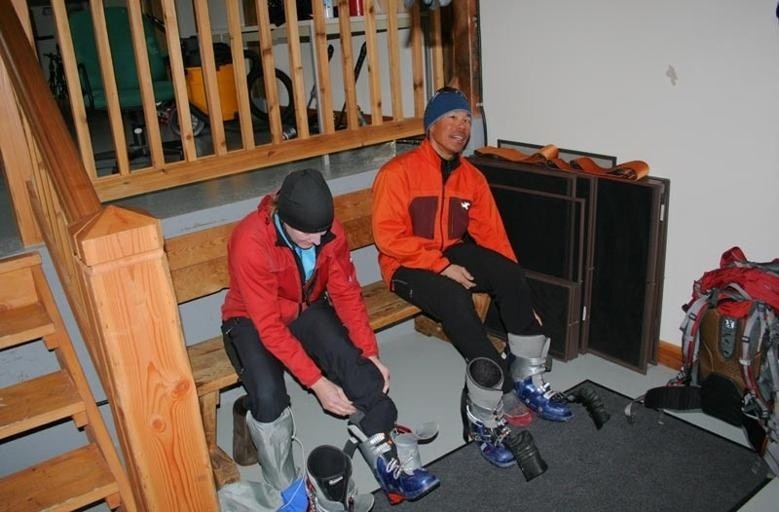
[508,332,573,421]
[467,357,518,467]
[308,446,374,512]
[346,412,439,505]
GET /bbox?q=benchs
[165,187,507,492]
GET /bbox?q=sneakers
[232,396,257,465]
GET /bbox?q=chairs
[57,5,186,173]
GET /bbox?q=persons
[221,168,440,512]
[370,84,576,427]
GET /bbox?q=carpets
[371,379,774,512]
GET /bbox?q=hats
[422,87,472,131]
[279,170,332,233]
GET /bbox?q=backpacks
[644,248,777,474]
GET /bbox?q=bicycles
[42,51,94,132]
